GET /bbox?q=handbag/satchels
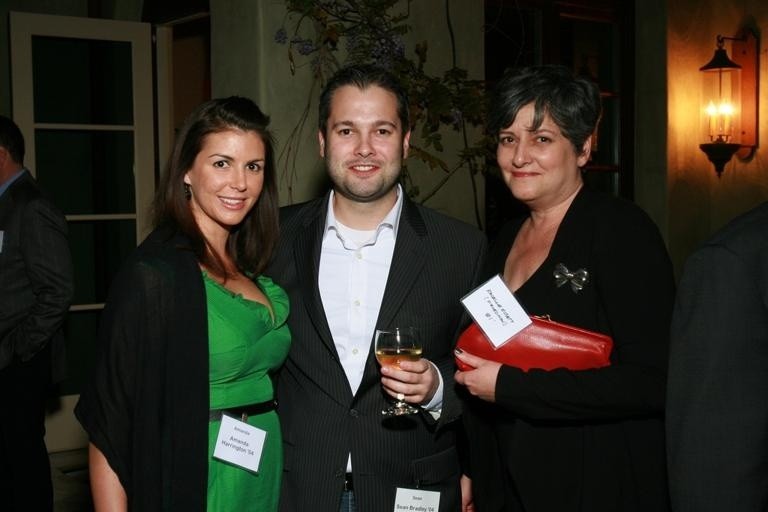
[454,315,612,374]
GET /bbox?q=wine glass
[375,327,422,417]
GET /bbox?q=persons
[662,198,765,510]
[0,117,83,510]
[447,60,678,510]
[70,93,294,512]
[245,61,492,510]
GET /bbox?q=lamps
[697,30,759,181]
